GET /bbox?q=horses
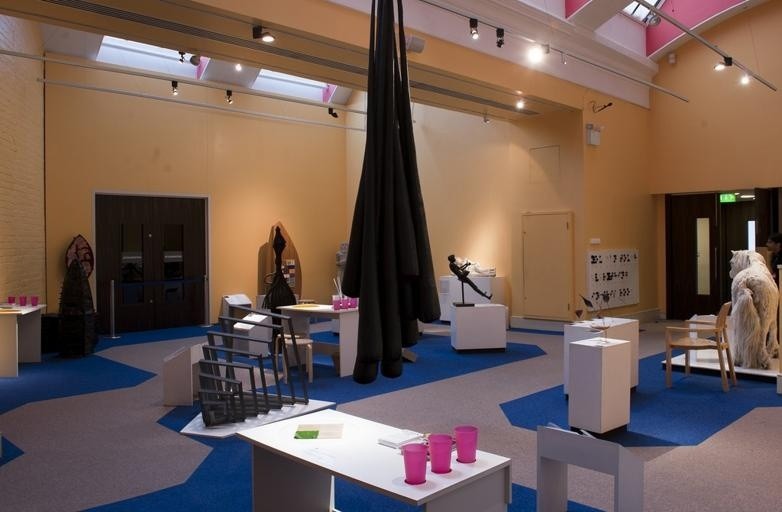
[727,249,779,371]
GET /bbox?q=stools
[282,340,313,385]
[275,333,309,381]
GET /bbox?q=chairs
[666,301,737,392]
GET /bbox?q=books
[378,427,457,460]
[296,422,347,440]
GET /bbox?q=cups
[7,296,38,307]
[400,424,480,486]
[332,293,358,310]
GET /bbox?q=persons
[766,232,782,285]
[448,255,493,300]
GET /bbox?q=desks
[236,408,513,511]
[277,305,359,375]
[0,304,49,377]
